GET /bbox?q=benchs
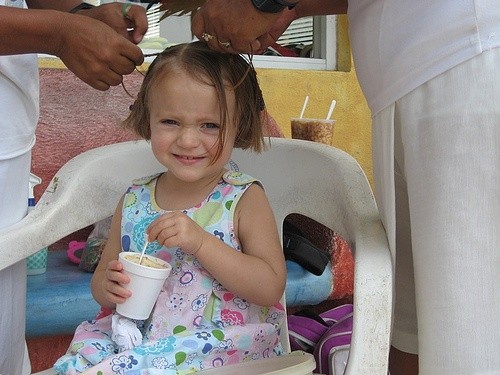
[26,65,336,336]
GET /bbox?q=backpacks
[287,303,354,375]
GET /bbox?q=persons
[50,42,285,375]
[191,0,500,374]
[0,0,149,375]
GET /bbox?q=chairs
[0,139,393,375]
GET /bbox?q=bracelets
[68,2,95,14]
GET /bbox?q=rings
[218,40,231,48]
[202,32,216,41]
[122,3,131,17]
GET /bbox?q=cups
[115,252,172,320]
[290,117,336,146]
